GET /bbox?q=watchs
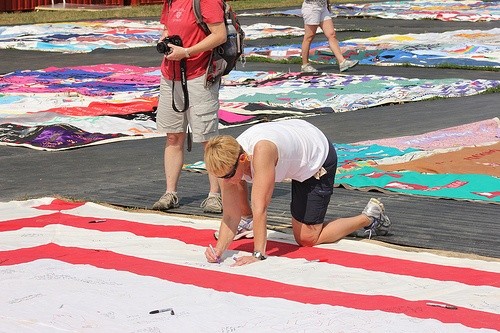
[253,251,267,260]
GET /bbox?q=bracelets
[185,48,190,59]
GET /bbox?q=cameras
[156,34,183,53]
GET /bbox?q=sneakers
[301,63,319,73]
[215,215,254,240]
[362,197,390,235]
[154,191,180,210]
[201,192,222,213]
[339,59,359,72]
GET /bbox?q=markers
[88,220,106,223]
[209,244,220,265]
[150,308,172,314]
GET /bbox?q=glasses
[218,145,243,180]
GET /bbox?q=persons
[204,119,392,267]
[301,0,359,72]
[152,0,227,213]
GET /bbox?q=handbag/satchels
[194,0,242,88]
